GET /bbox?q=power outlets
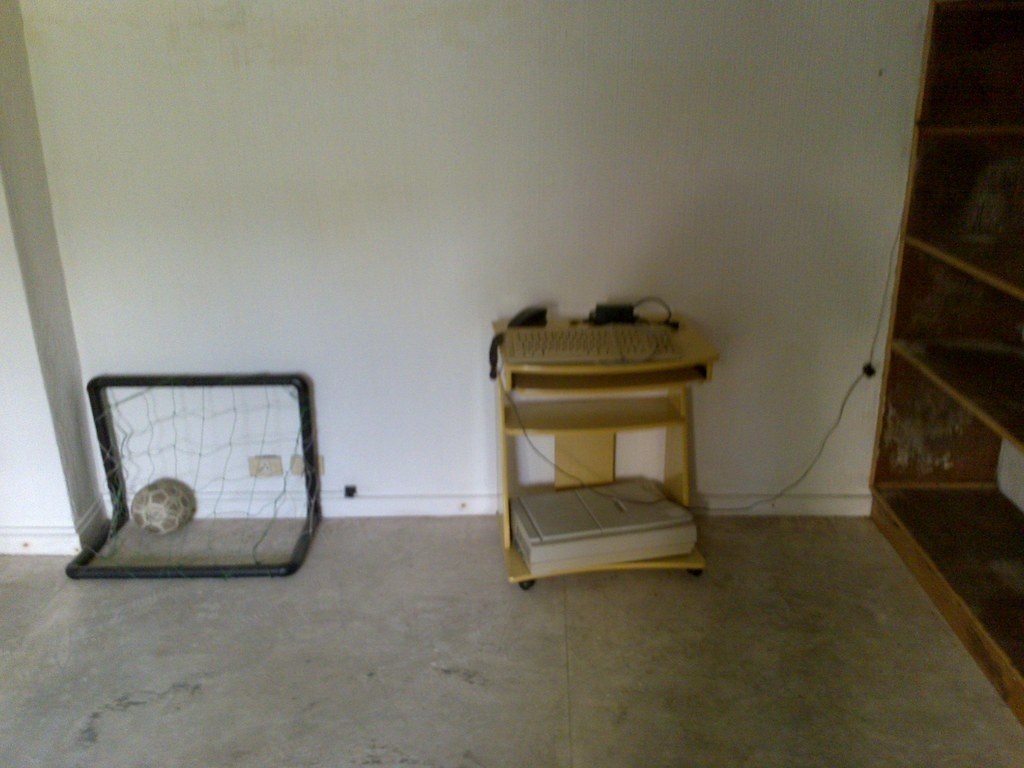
[247,454,284,478]
[290,455,325,476]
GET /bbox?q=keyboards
[503,324,685,363]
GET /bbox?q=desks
[491,317,721,593]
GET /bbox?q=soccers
[131,482,192,536]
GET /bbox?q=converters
[594,303,635,326]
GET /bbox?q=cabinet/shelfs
[867,0,1023,723]
